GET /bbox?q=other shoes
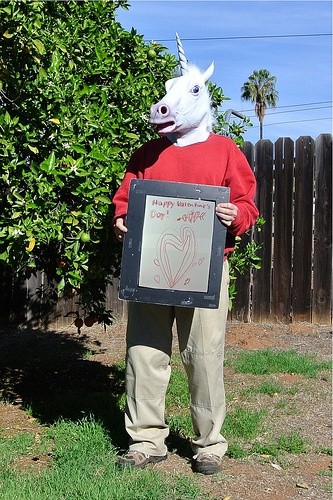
[117,450,168,469]
[192,452,223,475]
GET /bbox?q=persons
[113,33,259,473]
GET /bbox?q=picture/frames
[118,178,230,309]
